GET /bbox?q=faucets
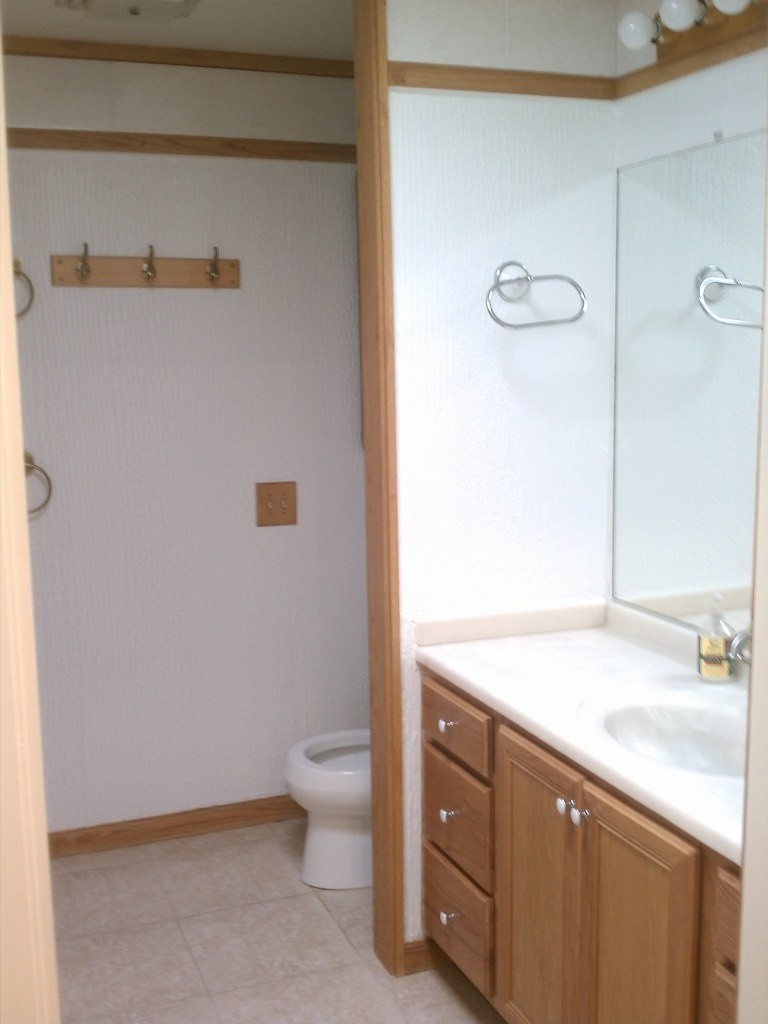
[726,629,753,667]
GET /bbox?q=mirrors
[617,129,764,643]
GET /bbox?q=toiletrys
[693,591,737,684]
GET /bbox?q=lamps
[617,0,751,51]
[55,0,203,26]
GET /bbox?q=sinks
[600,701,748,786]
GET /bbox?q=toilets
[281,726,373,892]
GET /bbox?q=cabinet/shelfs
[418,671,742,1023]
